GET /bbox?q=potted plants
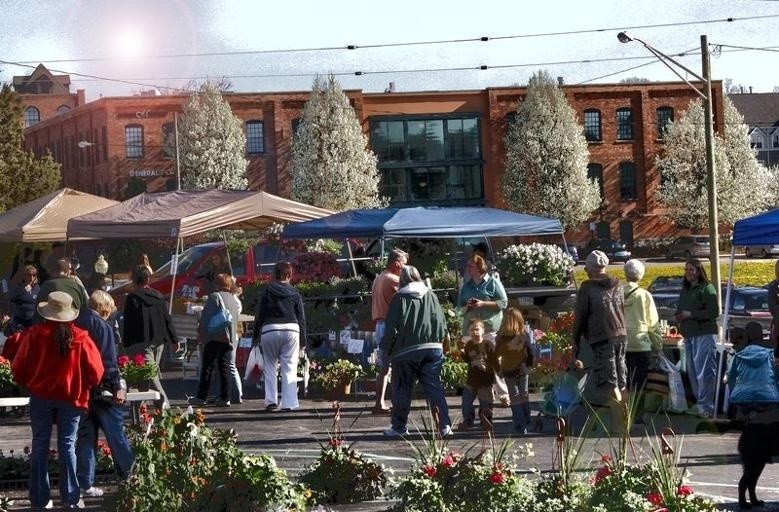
[311,359,364,396]
[529,367,544,395]
[442,347,469,394]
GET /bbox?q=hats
[585,250,608,273]
[36,290,79,323]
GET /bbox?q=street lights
[94,254,109,277]
[77,111,183,255]
[616,29,723,343]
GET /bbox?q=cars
[643,273,772,343]
[745,244,779,258]
[596,240,632,265]
[549,244,583,267]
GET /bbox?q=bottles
[660,320,671,335]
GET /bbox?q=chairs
[182,344,202,377]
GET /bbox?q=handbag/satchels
[206,293,232,335]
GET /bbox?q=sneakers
[32,486,103,508]
[381,410,648,437]
[187,393,297,413]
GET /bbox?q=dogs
[695,420,779,509]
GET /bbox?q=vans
[665,235,710,261]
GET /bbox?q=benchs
[1,388,160,431]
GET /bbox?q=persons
[371,248,536,439]
[252,261,307,409]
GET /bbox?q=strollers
[531,359,593,439]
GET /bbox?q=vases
[138,379,150,391]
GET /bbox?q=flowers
[679,485,694,495]
[129,496,138,506]
[330,437,342,446]
[424,464,435,478]
[648,493,668,512]
[133,355,144,364]
[140,404,160,424]
[160,442,168,450]
[197,478,206,485]
[49,448,57,455]
[173,417,180,424]
[195,409,205,426]
[118,355,128,366]
[441,452,462,468]
[491,464,508,483]
[129,477,138,486]
[98,440,111,455]
[190,492,196,502]
[191,459,198,466]
[537,357,568,374]
[24,446,30,454]
[10,449,14,454]
[595,456,613,480]
[304,489,310,498]
